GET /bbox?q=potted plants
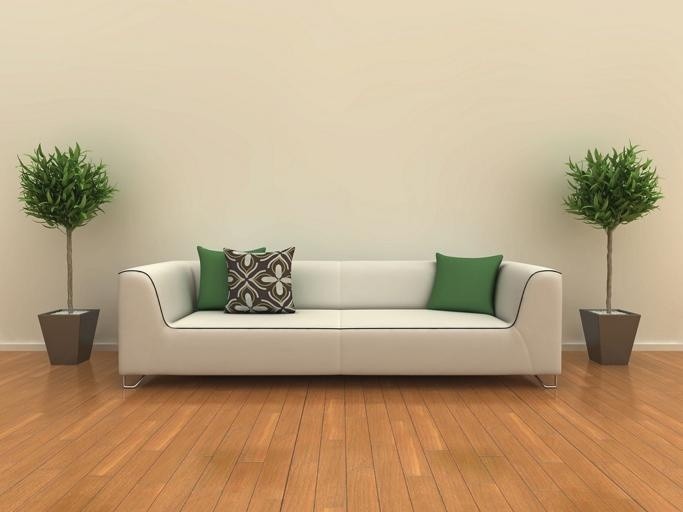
[561,138,664,365]
[14,141,120,366]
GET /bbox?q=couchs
[115,260,564,389]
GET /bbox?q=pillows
[224,247,297,313]
[427,252,504,315]
[197,245,266,311]
